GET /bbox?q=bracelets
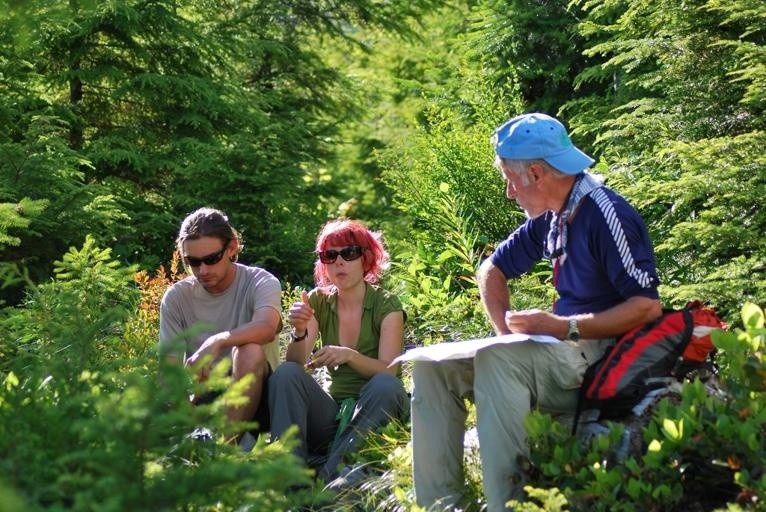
[291,328,309,342]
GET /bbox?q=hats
[496,111,596,177]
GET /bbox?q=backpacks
[572,303,732,463]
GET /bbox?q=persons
[270,220,409,488]
[159,205,285,451]
[411,111,661,512]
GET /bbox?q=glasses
[184,240,229,266]
[318,246,367,265]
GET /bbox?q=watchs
[566,316,580,341]
